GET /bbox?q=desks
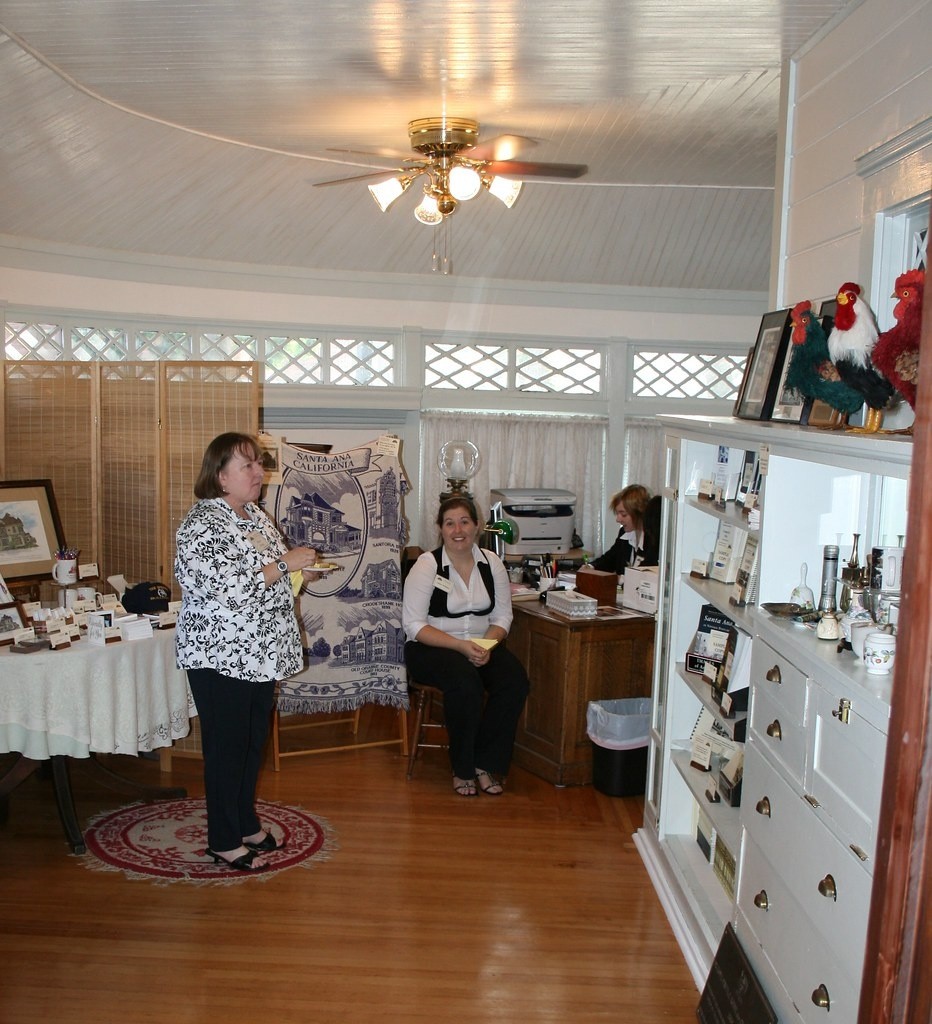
[0,627,199,855]
[504,600,657,790]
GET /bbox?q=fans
[311,117,589,190]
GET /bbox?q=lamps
[437,439,480,504]
[368,166,522,225]
[483,518,519,545]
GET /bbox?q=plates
[760,602,802,617]
[301,566,336,571]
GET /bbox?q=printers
[490,488,576,555]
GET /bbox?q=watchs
[274,559,289,575]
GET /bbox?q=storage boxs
[685,629,722,675]
[709,679,749,713]
[575,569,618,604]
[719,771,742,807]
[707,551,743,585]
[693,805,717,863]
[710,835,736,903]
[622,566,659,614]
[711,472,740,501]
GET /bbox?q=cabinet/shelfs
[632,411,912,1024]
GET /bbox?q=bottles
[871,547,883,590]
[817,609,838,640]
[840,601,874,642]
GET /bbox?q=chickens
[869,269,924,435]
[789,301,864,430]
[828,283,897,435]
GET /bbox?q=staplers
[539,585,565,603]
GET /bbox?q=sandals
[452,774,479,796]
[475,770,503,795]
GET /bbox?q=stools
[408,683,450,780]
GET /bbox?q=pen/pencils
[582,553,588,569]
[539,552,557,578]
[518,560,527,573]
[52,545,82,560]
[510,566,514,572]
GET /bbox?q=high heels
[242,832,285,851]
[205,847,269,872]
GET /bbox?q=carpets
[72,795,339,887]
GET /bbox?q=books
[713,628,752,701]
[689,704,745,786]
[690,803,735,903]
[734,531,759,604]
[693,601,734,660]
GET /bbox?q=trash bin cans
[585,698,653,797]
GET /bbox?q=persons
[399,491,531,798]
[175,430,327,872]
[578,483,655,591]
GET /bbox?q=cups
[864,633,896,675]
[52,560,76,584]
[77,587,103,609]
[851,623,892,665]
[58,589,77,608]
[881,548,903,591]
[540,577,558,593]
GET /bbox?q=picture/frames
[0,479,71,583]
[0,601,30,647]
[771,317,831,423]
[258,446,279,472]
[737,309,791,421]
[818,298,838,318]
